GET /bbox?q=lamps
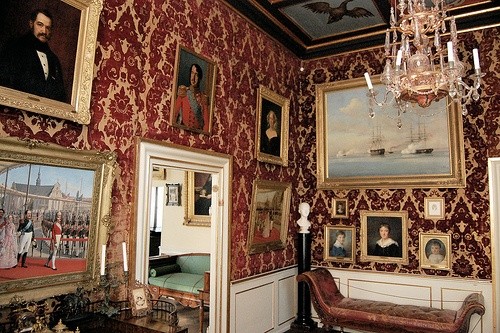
[363,0,487,129]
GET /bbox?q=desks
[198,289,210,333]
[13,312,164,333]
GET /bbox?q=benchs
[294,267,485,333]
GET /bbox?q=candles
[100,244,106,276]
[121,241,128,271]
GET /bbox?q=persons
[297,203,310,231]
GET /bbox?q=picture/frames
[128,285,150,317]
[171,43,218,137]
[255,84,291,169]
[0,136,116,306]
[0,0,104,125]
[424,197,445,221]
[330,196,348,218]
[183,169,211,227]
[153,166,165,181]
[315,65,467,189]
[247,179,292,256]
[359,210,410,265]
[165,183,180,206]
[324,225,357,263]
[419,233,452,271]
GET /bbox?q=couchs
[148,253,213,308]
